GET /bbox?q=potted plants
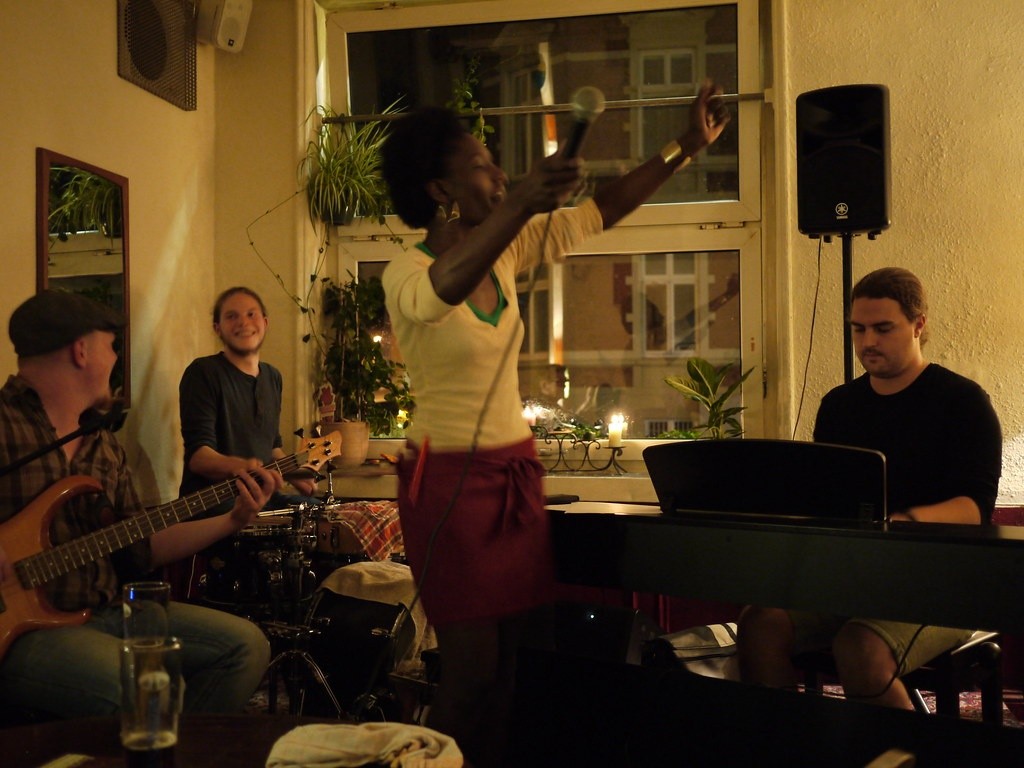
[315,276,413,467]
[296,91,416,221]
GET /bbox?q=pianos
[543,501,1023,636]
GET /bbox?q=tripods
[254,587,346,722]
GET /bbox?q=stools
[901,639,1003,724]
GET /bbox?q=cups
[118,637,183,750]
[123,581,170,646]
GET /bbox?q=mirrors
[37,147,132,409]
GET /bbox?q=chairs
[258,558,424,721]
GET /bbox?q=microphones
[562,87,606,160]
[103,401,128,432]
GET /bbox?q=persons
[735,268,1002,712]
[179,286,322,512]
[380,78,731,768]
[1,288,284,725]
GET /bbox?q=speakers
[795,85,891,242]
[196,0,253,53]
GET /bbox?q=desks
[548,495,1024,768]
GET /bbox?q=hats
[7,287,126,357]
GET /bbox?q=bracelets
[660,139,692,174]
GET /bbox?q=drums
[201,515,317,612]
[315,510,406,556]
[302,560,439,725]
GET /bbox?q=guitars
[0,427,345,662]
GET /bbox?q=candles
[608,415,624,446]
